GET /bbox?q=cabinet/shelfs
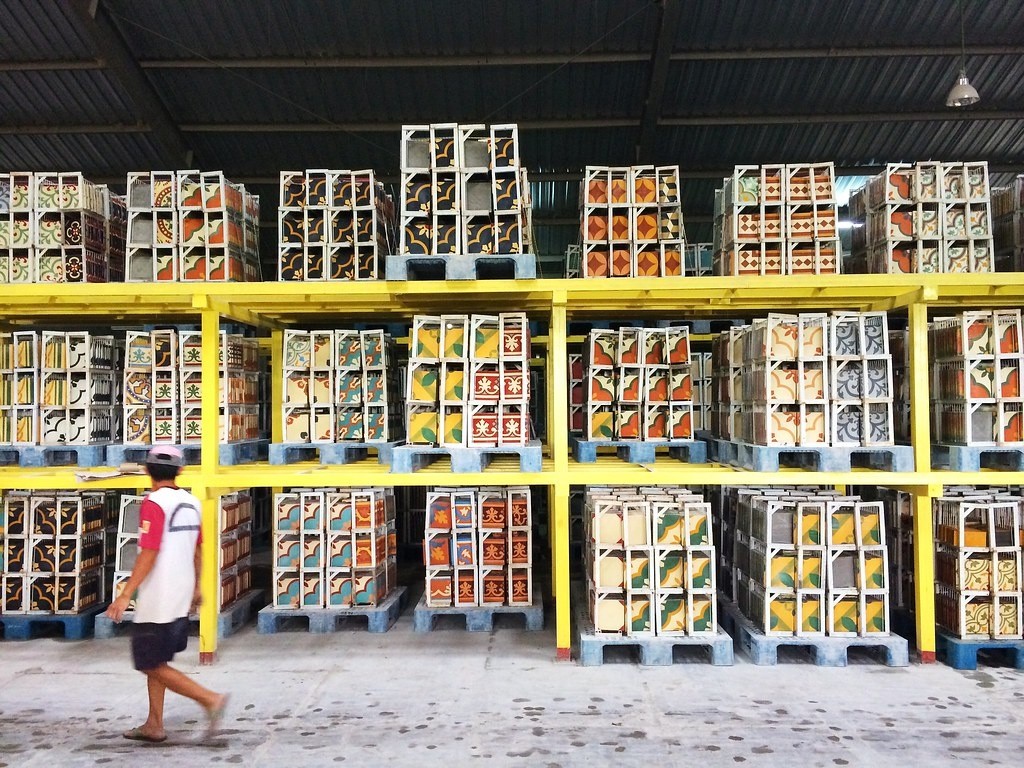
[0,273,1024,669]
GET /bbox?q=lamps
[946,0,979,106]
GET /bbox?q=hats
[145,446,187,466]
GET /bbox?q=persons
[106,446,228,741]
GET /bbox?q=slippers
[205,691,236,728]
[124,726,169,742]
